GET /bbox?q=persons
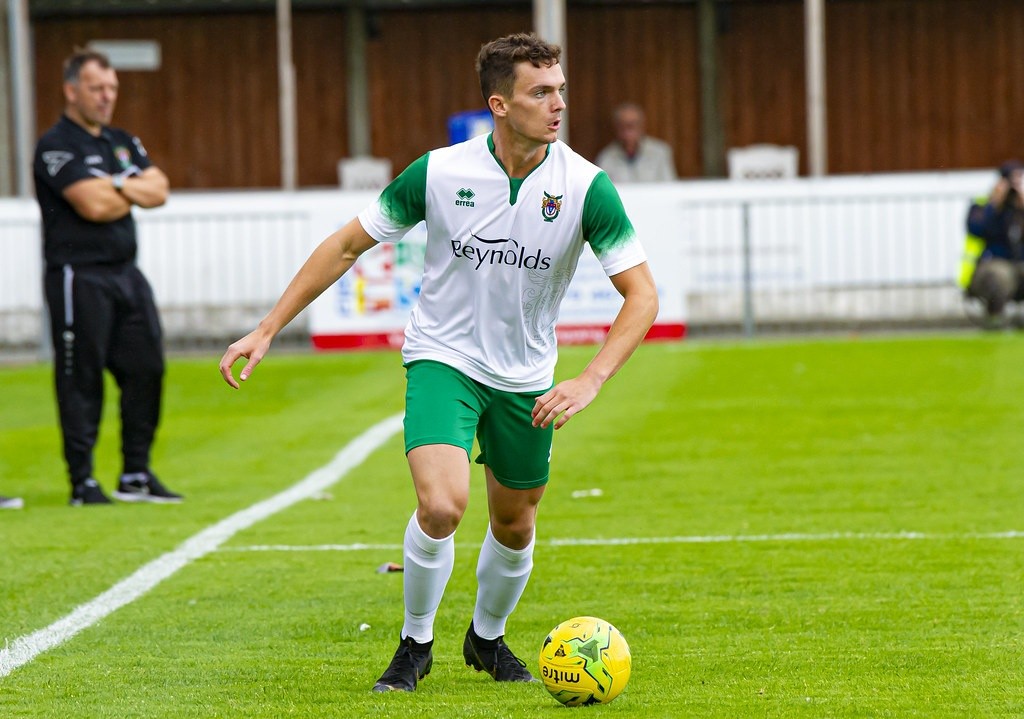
[32,50,183,505]
[595,103,680,184]
[964,162,1024,329]
[218,31,658,688]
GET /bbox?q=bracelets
[111,172,121,193]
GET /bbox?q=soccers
[537,615,633,707]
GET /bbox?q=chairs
[336,155,393,189]
[724,141,801,181]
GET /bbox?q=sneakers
[463,618,531,681]
[371,630,435,692]
[69,479,111,503]
[112,470,183,504]
